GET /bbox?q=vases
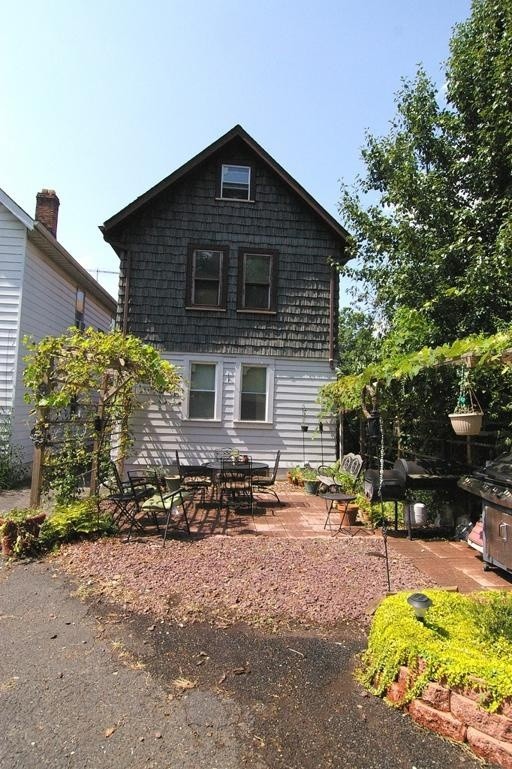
[337,503,359,526]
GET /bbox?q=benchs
[317,453,364,496]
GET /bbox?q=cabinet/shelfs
[482,499,511,575]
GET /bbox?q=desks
[321,491,355,536]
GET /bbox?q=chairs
[97,450,286,548]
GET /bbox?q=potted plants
[0,506,52,568]
[449,363,483,435]
[286,463,321,495]
[356,497,372,521]
